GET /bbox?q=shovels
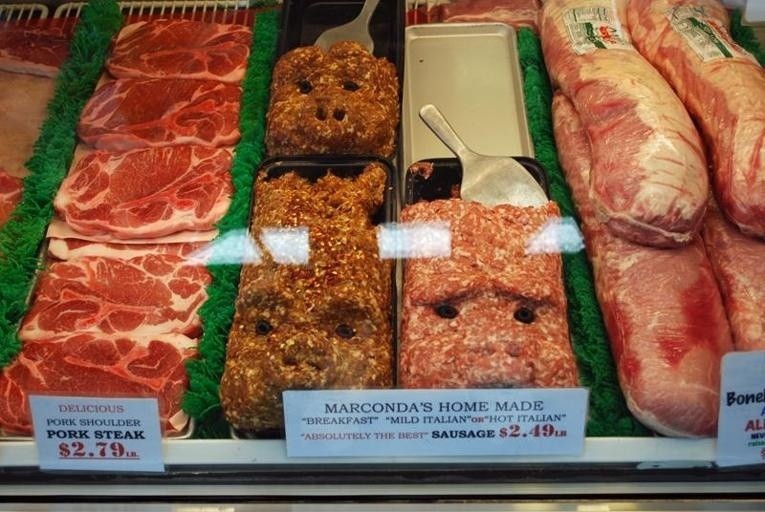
[419,103,550,208]
[312,0,381,54]
